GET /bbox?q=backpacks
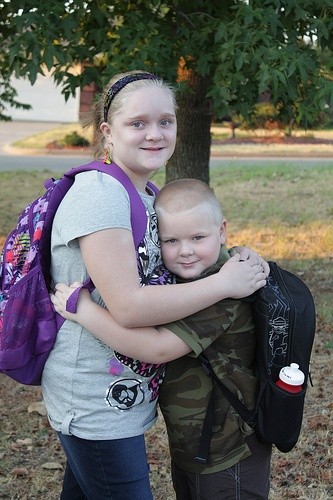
[188,260,316,474]
[0,161,160,386]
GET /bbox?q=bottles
[275,366,305,393]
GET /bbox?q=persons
[40,71,270,500]
[50,179,270,500]
